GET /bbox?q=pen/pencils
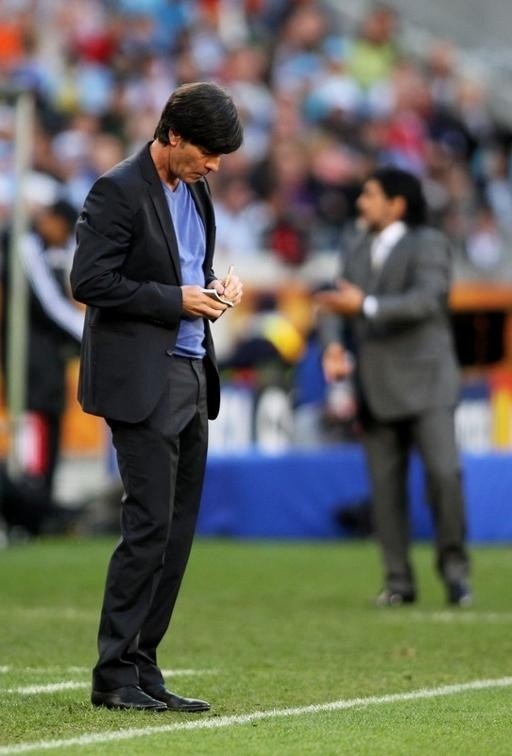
[221,266,232,298]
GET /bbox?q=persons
[71,82,243,712]
[309,167,474,607]
[3,1,511,397]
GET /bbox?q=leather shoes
[377,587,416,605]
[91,687,209,713]
[446,590,472,609]
